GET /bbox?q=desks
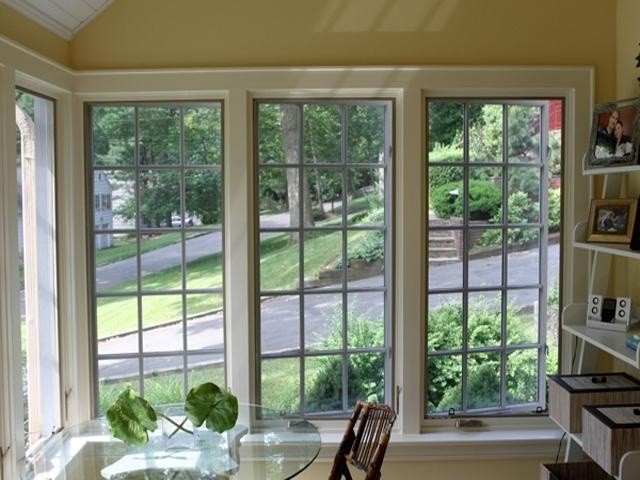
[20,403,321,480]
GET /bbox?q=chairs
[329,401,396,480]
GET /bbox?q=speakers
[586,295,632,333]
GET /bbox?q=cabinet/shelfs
[550,152,640,480]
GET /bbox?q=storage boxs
[540,460,618,480]
[581,404,640,477]
[546,371,640,433]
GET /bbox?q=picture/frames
[585,199,638,244]
[588,97,640,167]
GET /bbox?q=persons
[595,109,620,153]
[601,211,618,233]
[613,121,632,155]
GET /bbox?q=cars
[161,215,193,228]
